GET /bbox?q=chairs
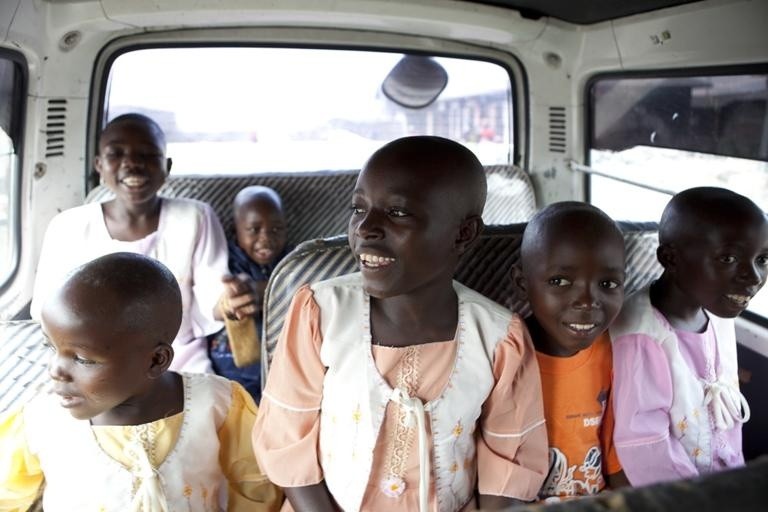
[0,318,58,419]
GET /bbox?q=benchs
[259,221,663,413]
[81,164,539,276]
[497,457,768,512]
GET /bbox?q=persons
[251,136,550,512]
[207,184,287,407]
[606,186,768,489]
[511,201,631,504]
[0,252,284,511]
[29,114,258,376]
